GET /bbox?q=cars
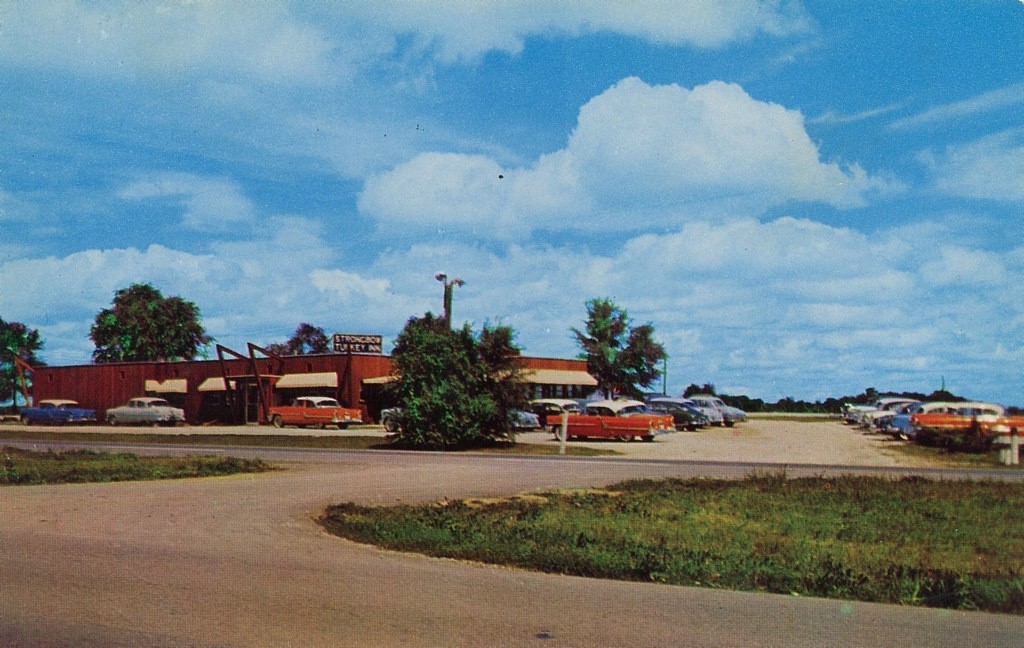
[545,401,677,441]
[886,401,1006,442]
[19,398,98,425]
[650,394,711,431]
[840,398,921,431]
[690,394,748,428]
[528,399,585,423]
[267,396,363,428]
[509,410,542,429]
[105,398,186,425]
[911,414,1024,451]
[688,398,723,428]
[381,407,404,433]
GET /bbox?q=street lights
[434,272,467,330]
